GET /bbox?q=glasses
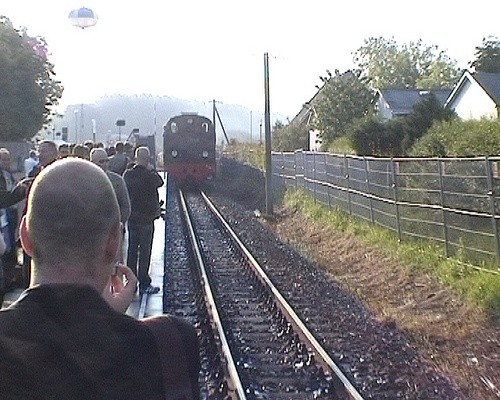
[98,159,109,163]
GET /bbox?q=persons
[122,146,164,294]
[0,138,131,293]
[0,157,200,400]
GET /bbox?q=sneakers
[139,285,160,294]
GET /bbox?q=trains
[162,112,218,186]
